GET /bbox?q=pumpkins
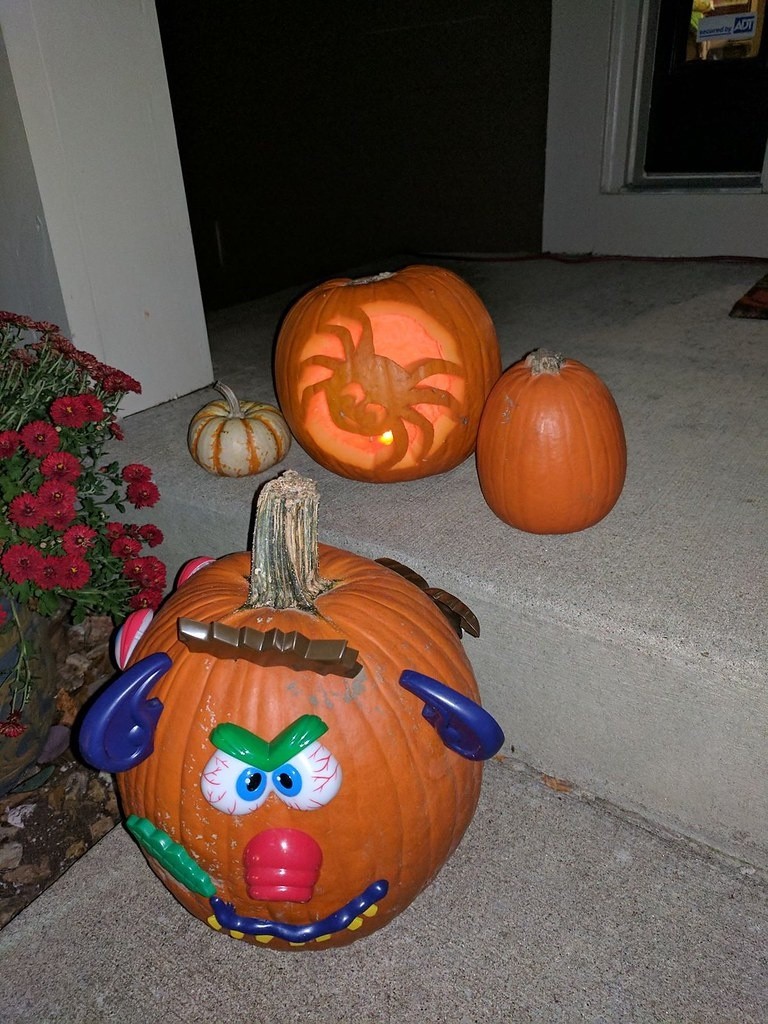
[188,381,292,477]
[111,469,481,948]
[478,345,627,534]
